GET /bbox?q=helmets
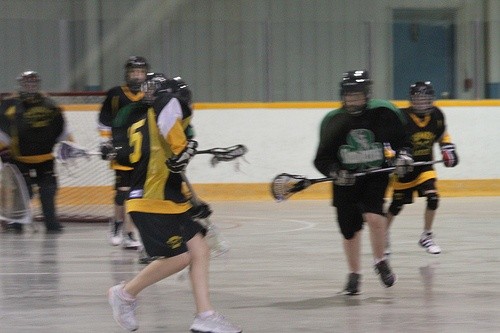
[124,56,150,93]
[18,71,43,99]
[340,69,374,115]
[154,75,193,105]
[140,72,168,104]
[409,81,435,116]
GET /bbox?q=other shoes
[7,222,22,232]
[45,222,64,231]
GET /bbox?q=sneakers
[108,282,140,331]
[189,312,243,333]
[375,258,398,288]
[344,272,362,295]
[122,232,143,248]
[418,231,441,254]
[112,217,124,246]
[384,239,391,254]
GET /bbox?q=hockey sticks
[181,170,234,253]
[270,165,397,200]
[414,159,446,165]
[54,141,105,165]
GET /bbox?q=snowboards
[195,144,247,162]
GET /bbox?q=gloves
[164,141,196,173]
[328,168,357,186]
[186,196,213,220]
[101,142,122,161]
[441,143,458,167]
[392,150,414,175]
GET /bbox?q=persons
[0,71,77,233]
[384,82,458,256]
[96,55,150,249]
[107,74,243,333]
[313,70,417,295]
[110,73,208,263]
